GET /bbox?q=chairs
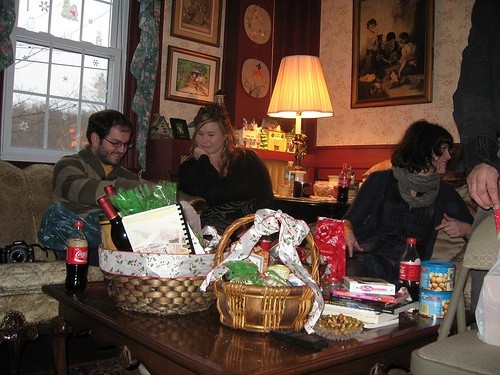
[410,212,500,375]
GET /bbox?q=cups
[418,260,456,319]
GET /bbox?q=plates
[245,5,271,43]
[241,60,269,98]
[311,315,363,340]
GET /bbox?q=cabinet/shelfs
[256,150,316,196]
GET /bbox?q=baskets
[98,245,217,314]
[213,209,320,334]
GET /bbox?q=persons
[454,0,500,331]
[367,17,418,88]
[53,110,158,268]
[177,103,276,246]
[341,121,474,292]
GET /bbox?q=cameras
[0,240,34,263]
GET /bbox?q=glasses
[103,137,134,148]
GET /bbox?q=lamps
[268,55,335,197]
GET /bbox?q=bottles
[65,221,88,293]
[398,237,421,302]
[104,184,122,218]
[283,160,295,196]
[97,195,133,252]
[337,162,350,203]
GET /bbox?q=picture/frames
[164,45,220,105]
[170,117,190,139]
[351,0,433,108]
[171,0,223,47]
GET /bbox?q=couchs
[0,161,205,375]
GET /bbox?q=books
[320,276,420,329]
[121,203,194,254]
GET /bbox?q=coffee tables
[43,277,476,375]
